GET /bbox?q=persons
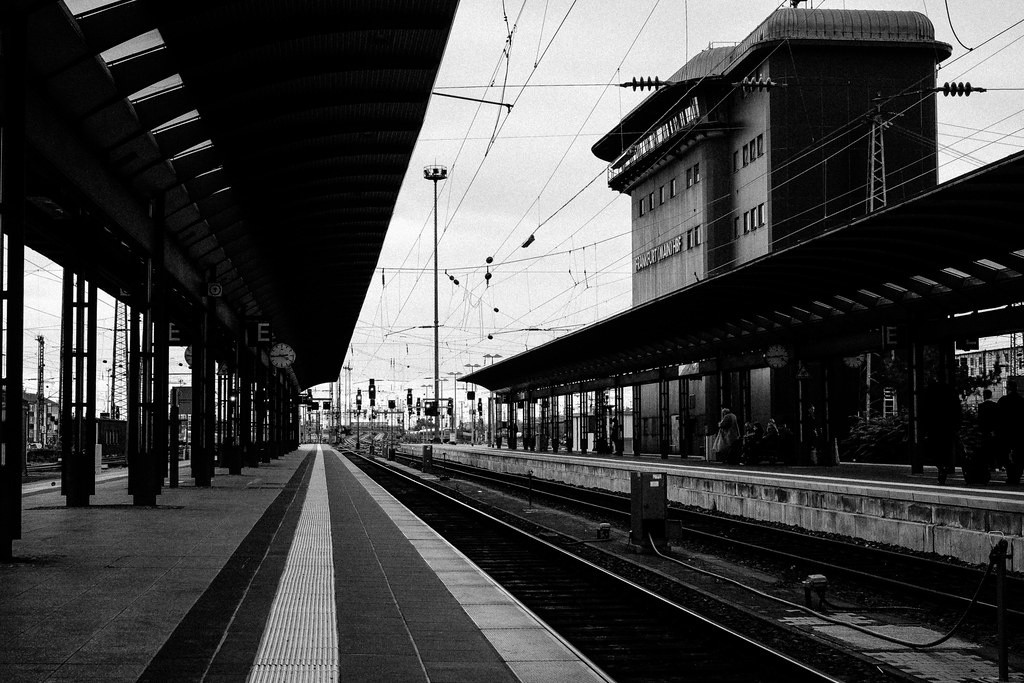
[976,380,1024,485]
[718,408,740,463]
[806,404,822,464]
